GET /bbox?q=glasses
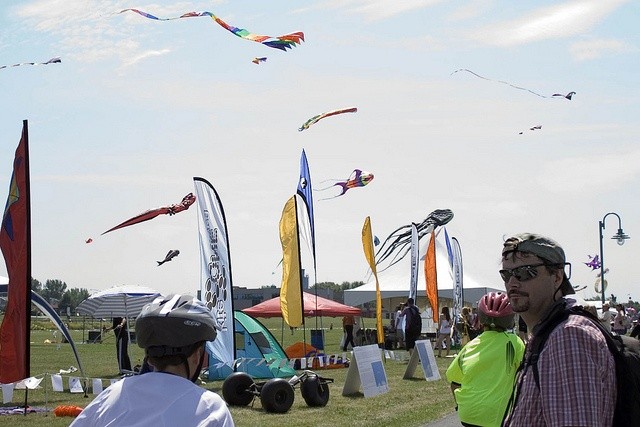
[499,263,554,283]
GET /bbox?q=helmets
[478,290,514,328]
[136,293,217,347]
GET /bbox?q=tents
[210,309,298,381]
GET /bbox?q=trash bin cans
[310,329,325,351]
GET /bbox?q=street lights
[599,212,630,312]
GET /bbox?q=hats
[502,231,576,295]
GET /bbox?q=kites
[298,104,358,134]
[0,57,63,70]
[530,123,543,134]
[372,237,380,246]
[85,193,196,243]
[584,253,601,273]
[156,249,179,266]
[365,208,454,285]
[110,8,305,66]
[583,253,601,270]
[552,90,576,102]
[312,169,374,201]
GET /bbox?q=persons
[399,298,423,358]
[68,291,235,426]
[395,307,404,349]
[600,303,612,332]
[499,232,618,427]
[446,292,525,427]
[613,304,627,335]
[462,307,480,338]
[436,308,451,357]
[342,315,356,351]
[104,317,132,377]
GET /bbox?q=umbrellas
[74,285,161,378]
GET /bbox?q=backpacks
[409,306,422,332]
[531,305,639,426]
